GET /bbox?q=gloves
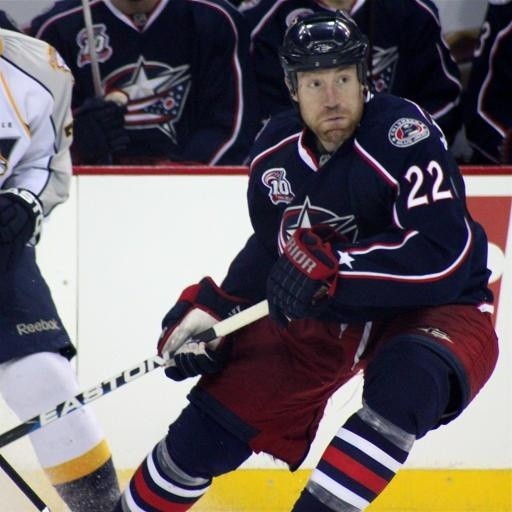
[74,97,124,148]
[0,189,44,257]
[158,277,251,380]
[268,225,348,328]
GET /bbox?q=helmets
[280,9,369,94]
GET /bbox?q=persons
[0,0,512,166]
[0,28,121,512]
[121,8,500,512]
